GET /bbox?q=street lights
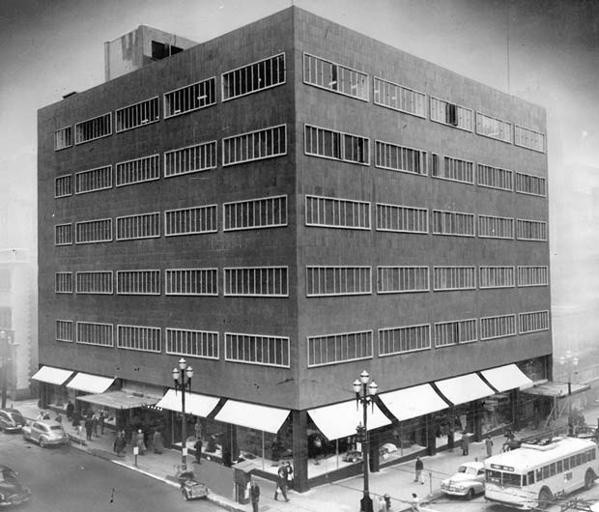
[558,348,581,439]
[0,325,21,408]
[170,355,195,479]
[352,368,379,512]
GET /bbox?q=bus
[484,433,598,512]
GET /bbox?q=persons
[93,415,99,438]
[274,470,290,502]
[43,411,50,419]
[152,429,164,455]
[485,435,493,457]
[384,493,392,512]
[113,428,127,456]
[37,411,43,419]
[193,437,203,464]
[55,412,63,423]
[136,428,146,455]
[278,462,287,484]
[98,412,104,435]
[248,480,260,512]
[66,400,74,421]
[85,418,93,441]
[462,432,468,456]
[408,493,420,512]
[286,461,294,489]
[414,456,424,485]
[71,410,83,434]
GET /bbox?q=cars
[19,419,70,450]
[438,460,485,501]
[0,463,33,511]
[0,406,29,434]
